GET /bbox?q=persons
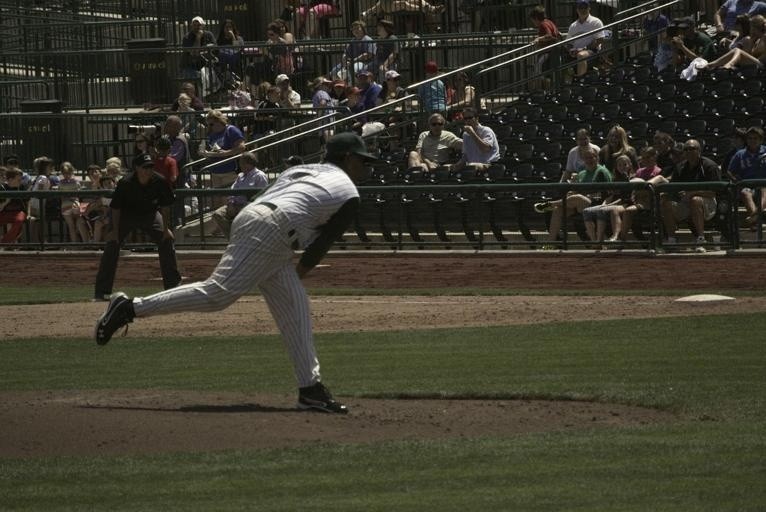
[95,155,181,302]
[528,1,606,91]
[643,0,766,82]
[93,133,377,413]
[542,125,764,253]
[1,1,501,254]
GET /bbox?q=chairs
[344,25,766,226]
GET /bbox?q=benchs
[120,31,549,136]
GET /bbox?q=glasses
[464,116,473,119]
[431,123,443,126]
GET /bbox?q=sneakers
[695,236,706,253]
[298,381,349,415]
[94,291,134,346]
[534,200,555,214]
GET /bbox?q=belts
[255,201,299,250]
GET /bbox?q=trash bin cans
[219,0,262,41]
[127,39,171,105]
[20,100,66,169]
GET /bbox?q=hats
[326,132,379,162]
[275,61,438,97]
[134,152,155,167]
